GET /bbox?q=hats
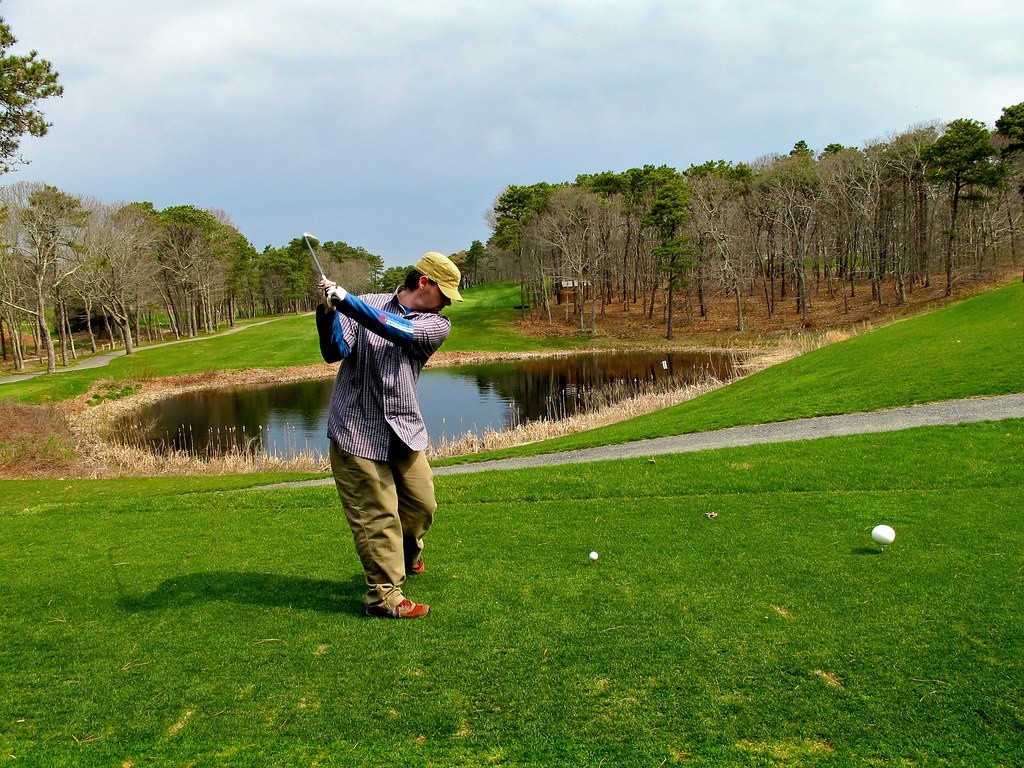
[414,252,464,303]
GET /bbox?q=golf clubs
[300,232,343,312]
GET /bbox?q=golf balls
[872,525,896,544]
[589,551,599,560]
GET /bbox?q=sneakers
[409,559,425,573]
[363,598,430,618]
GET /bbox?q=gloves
[325,286,347,311]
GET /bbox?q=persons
[316,252,464,618]
